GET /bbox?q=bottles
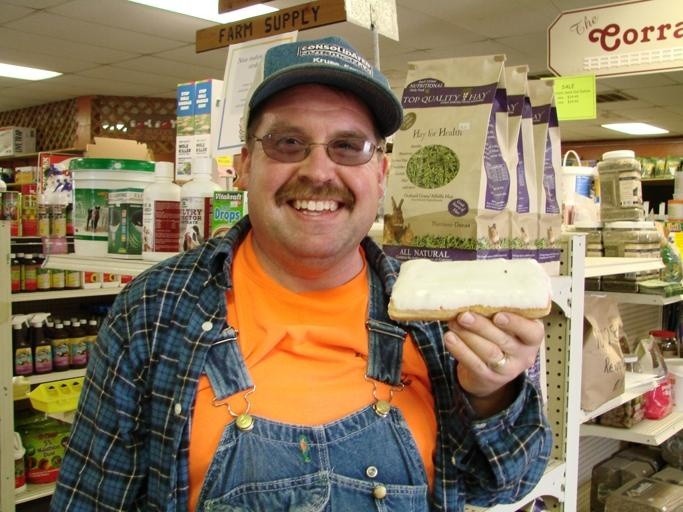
[1,192,135,379]
[598,326,677,432]
[179,156,223,257]
[570,149,683,301]
[141,159,180,263]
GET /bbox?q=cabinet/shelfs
[579,253,682,447]
[35,250,578,511]
[0,220,141,512]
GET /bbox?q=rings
[487,353,507,371]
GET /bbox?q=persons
[50,36,553,512]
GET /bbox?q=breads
[387,258,553,322]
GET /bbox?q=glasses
[251,130,384,166]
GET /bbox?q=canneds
[0,191,74,236]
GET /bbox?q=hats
[245,33,402,141]
